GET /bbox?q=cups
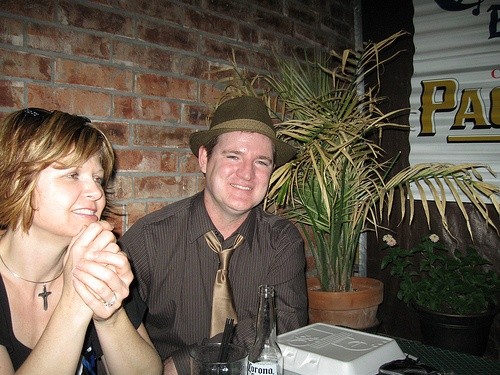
[189,343,248,375]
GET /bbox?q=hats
[190,96,298,169]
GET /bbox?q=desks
[380,333,500,375]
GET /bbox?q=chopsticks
[215,318,234,370]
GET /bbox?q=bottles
[248,284,283,375]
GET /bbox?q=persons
[0,107,164,375]
[80,96,306,375]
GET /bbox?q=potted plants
[203,29,500,329]
[416,312,496,357]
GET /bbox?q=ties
[204,230,245,338]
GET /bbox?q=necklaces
[0,238,63,311]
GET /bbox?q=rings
[103,293,116,307]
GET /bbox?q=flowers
[377,234,500,312]
